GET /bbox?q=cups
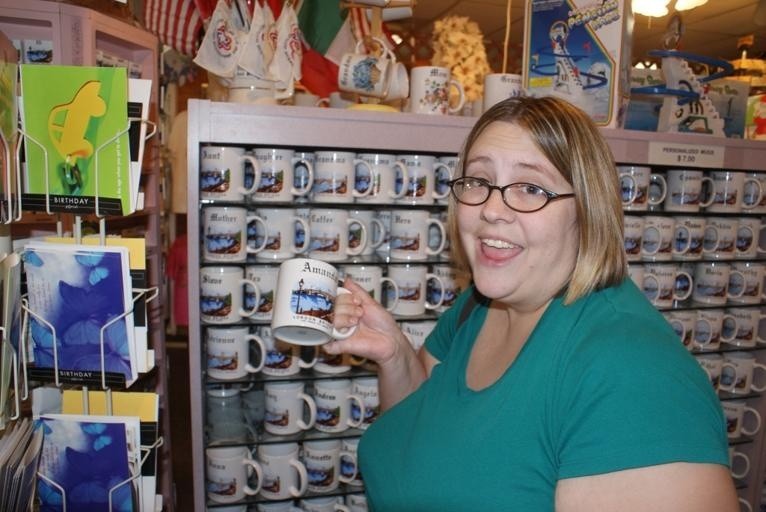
[309,208,367,261]
[339,265,399,312]
[295,209,310,258]
[616,167,638,204]
[246,211,255,257]
[199,266,263,323]
[410,66,465,117]
[706,172,764,212]
[727,307,766,348]
[729,262,766,304]
[303,439,358,493]
[721,351,766,395]
[355,154,410,204]
[672,268,694,307]
[742,174,766,213]
[671,311,714,352]
[340,437,367,487]
[738,497,754,512]
[359,48,409,104]
[401,321,439,356]
[263,380,318,436]
[201,324,266,380]
[703,218,756,260]
[429,213,441,247]
[694,310,740,349]
[261,326,321,376]
[722,401,763,439]
[257,442,309,500]
[206,504,248,511]
[628,265,661,305]
[299,496,352,511]
[693,263,746,305]
[205,397,254,433]
[694,354,739,396]
[204,374,258,396]
[619,167,668,211]
[294,152,316,202]
[395,156,453,204]
[245,265,280,321]
[244,151,254,200]
[374,211,392,252]
[359,322,415,372]
[644,265,693,307]
[735,218,766,259]
[388,266,445,316]
[659,312,686,344]
[199,208,268,263]
[642,216,692,262]
[207,418,257,455]
[348,210,386,256]
[256,500,304,511]
[243,390,274,441]
[648,173,666,211]
[432,264,462,312]
[437,156,461,205]
[313,378,366,433]
[271,257,360,346]
[200,146,262,203]
[251,148,314,202]
[205,438,264,503]
[312,151,374,202]
[255,208,312,259]
[439,212,450,249]
[664,170,716,211]
[345,493,369,511]
[313,344,367,373]
[482,73,522,114]
[672,217,722,260]
[624,216,663,261]
[729,445,752,479]
[338,37,390,96]
[391,210,452,260]
[352,377,381,429]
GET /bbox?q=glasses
[446,176,575,213]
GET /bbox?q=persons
[325,95,741,512]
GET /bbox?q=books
[0,416,42,512]
[1,252,23,431]
[1,30,18,197]
[37,388,162,511]
[25,236,153,386]
[19,64,152,215]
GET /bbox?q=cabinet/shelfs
[0,1,170,512]
[186,100,765,512]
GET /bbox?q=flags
[144,0,200,59]
[195,3,303,99]
